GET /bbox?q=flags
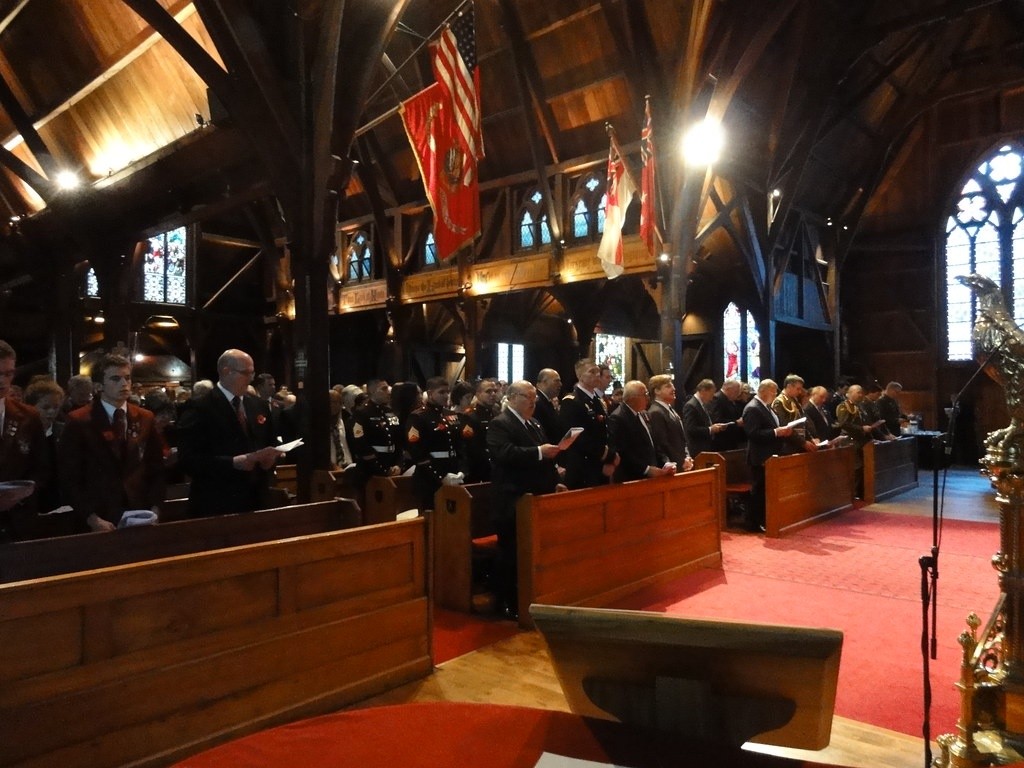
[598,99,656,279]
[398,2,486,266]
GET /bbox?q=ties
[113,409,126,456]
[593,397,604,415]
[232,396,247,432]
[526,422,541,443]
[793,400,803,417]
[638,414,653,447]
[331,424,347,469]
[669,407,675,414]
[704,406,714,440]
[769,406,779,426]
[819,407,828,426]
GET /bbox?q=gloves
[443,472,465,486]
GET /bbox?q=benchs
[707,449,754,497]
[0,497,363,584]
[433,482,499,615]
[365,475,426,526]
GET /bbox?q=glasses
[635,392,649,398]
[514,393,538,403]
[236,370,255,381]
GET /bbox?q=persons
[0,341,911,622]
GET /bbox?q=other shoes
[756,525,766,533]
[502,604,518,620]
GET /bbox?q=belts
[372,445,395,453]
[430,450,457,458]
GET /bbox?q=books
[786,416,806,429]
[557,426,584,451]
[871,420,885,428]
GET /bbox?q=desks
[172,703,848,768]
[902,434,961,470]
